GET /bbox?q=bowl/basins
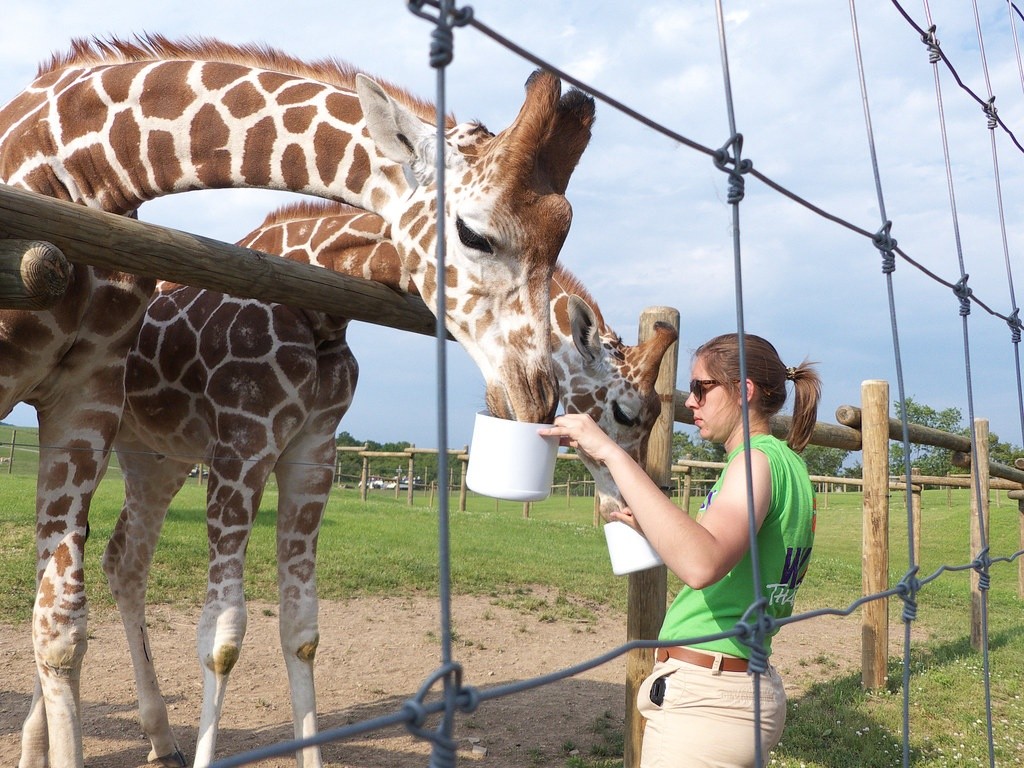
[603,520,664,575]
[465,408,561,503]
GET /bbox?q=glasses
[689,379,741,403]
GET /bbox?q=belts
[655,646,750,672]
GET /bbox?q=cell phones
[650,677,666,706]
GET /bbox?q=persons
[555,334,822,768]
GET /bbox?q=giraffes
[21,203,680,768]
[0,32,594,768]
